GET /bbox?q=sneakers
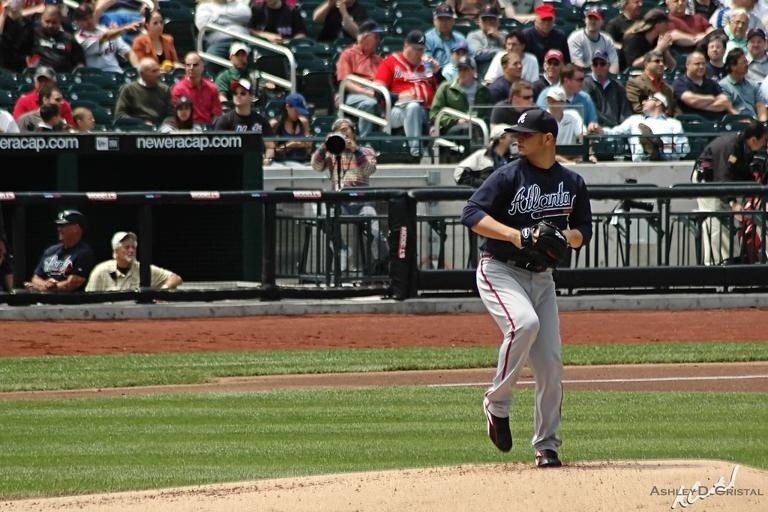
[536,450,561,465]
[487,410,512,451]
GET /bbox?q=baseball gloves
[521,221,570,264]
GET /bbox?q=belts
[493,254,545,273]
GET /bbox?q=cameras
[748,151,768,172]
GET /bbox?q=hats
[33,3,765,138]
[54,209,84,224]
[112,231,138,247]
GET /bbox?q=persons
[24,210,92,293]
[453,124,514,189]
[85,231,182,292]
[0,0,768,157]
[0,233,15,294]
[310,119,381,278]
[692,124,766,266]
[462,110,592,466]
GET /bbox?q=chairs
[0,0,768,159]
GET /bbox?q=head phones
[352,123,360,136]
